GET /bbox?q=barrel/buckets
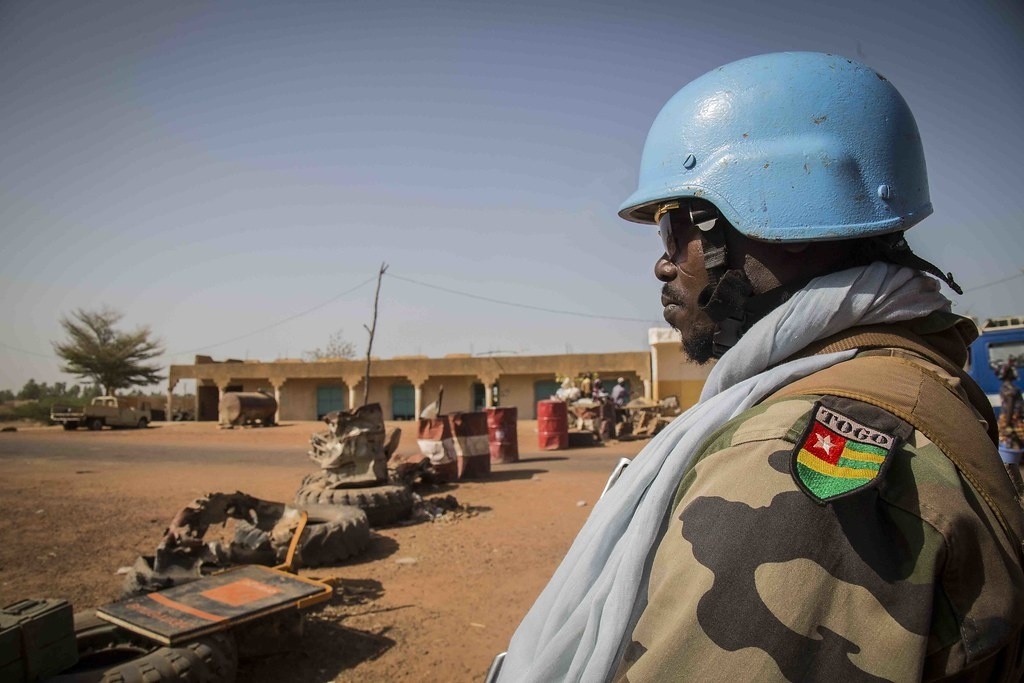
[482,407,520,465]
[535,400,569,451]
[415,416,461,484]
[448,411,492,478]
[575,404,602,441]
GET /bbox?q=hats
[617,377,625,384]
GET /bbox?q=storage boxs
[0,598,78,683]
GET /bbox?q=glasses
[654,200,712,261]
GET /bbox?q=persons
[994,364,1024,448]
[580,370,635,440]
[479,49,1024,682]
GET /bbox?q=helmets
[616,53,933,242]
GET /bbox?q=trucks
[50,396,151,431]
